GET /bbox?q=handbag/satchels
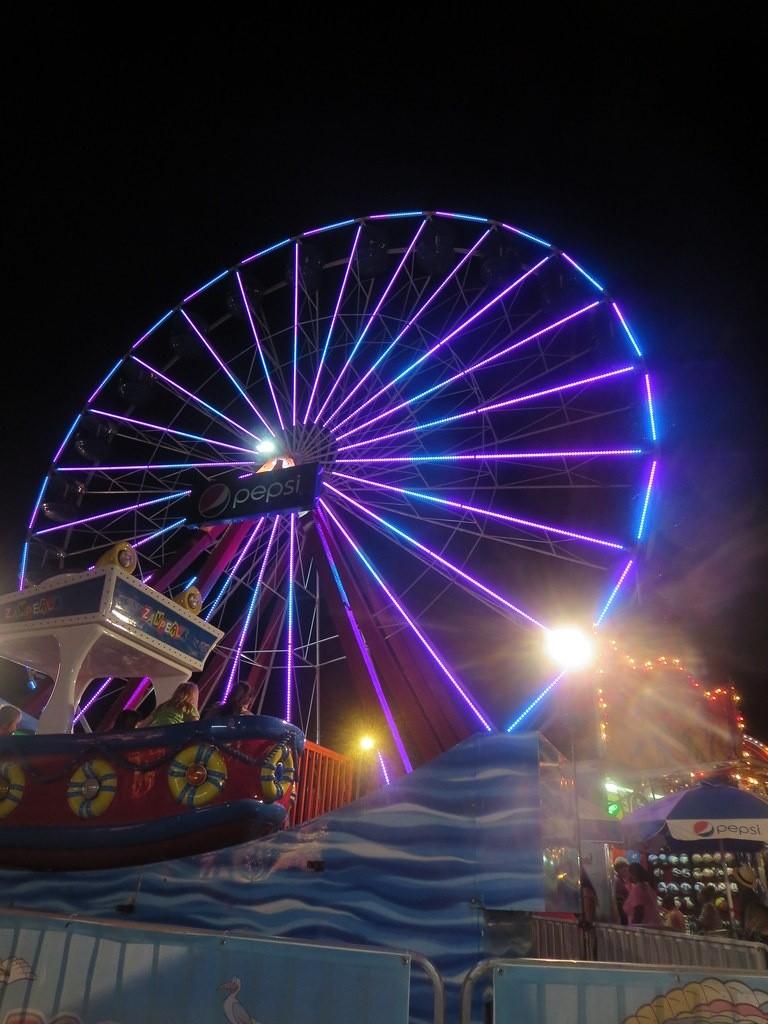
[633,905,645,924]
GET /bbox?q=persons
[614,856,685,933]
[692,887,724,936]
[580,863,600,923]
[733,864,768,935]
[0,704,21,736]
[104,709,141,732]
[204,681,255,719]
[137,682,200,729]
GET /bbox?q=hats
[680,882,692,893]
[647,851,737,868]
[653,868,664,879]
[681,869,690,878]
[715,867,724,880]
[656,896,663,906]
[674,897,682,908]
[730,882,738,893]
[734,866,757,888]
[726,867,733,875]
[694,882,706,892]
[715,897,728,910]
[671,868,681,880]
[692,868,703,880]
[667,882,680,894]
[717,882,727,891]
[657,881,667,893]
[707,882,717,892]
[703,868,715,878]
[684,897,694,909]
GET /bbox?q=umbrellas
[538,772,624,844]
[615,778,768,936]
